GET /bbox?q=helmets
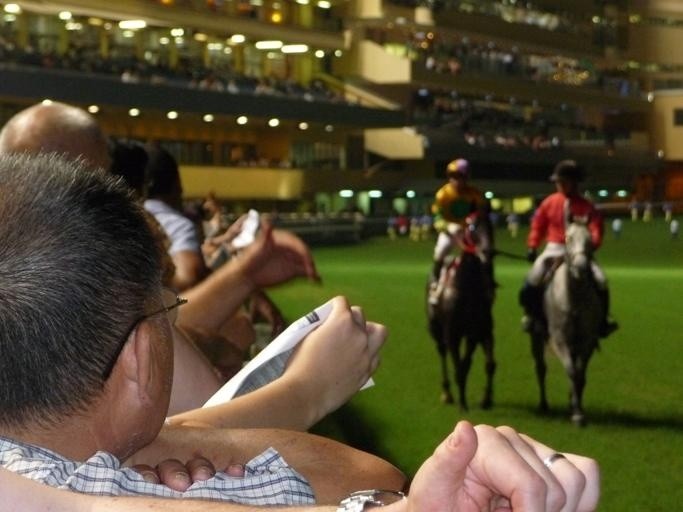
[447,158,474,176]
[549,159,576,183]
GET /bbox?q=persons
[0,99,606,512]
[1,2,375,231]
[374,0,680,240]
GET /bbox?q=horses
[424,201,497,414]
[526,209,605,429]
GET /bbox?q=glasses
[100,290,189,381]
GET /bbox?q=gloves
[526,248,537,262]
